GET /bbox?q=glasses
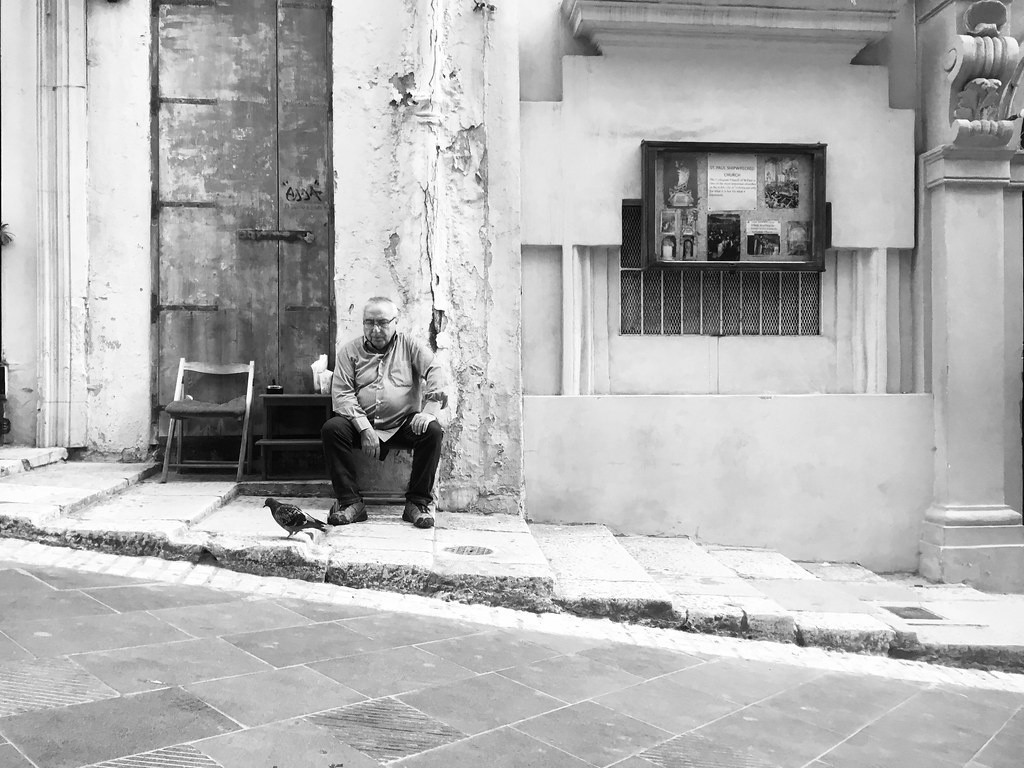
[363,317,396,327]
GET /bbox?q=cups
[319,375,332,394]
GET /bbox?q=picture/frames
[641,140,825,272]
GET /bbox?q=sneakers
[402,501,434,527]
[327,498,368,525]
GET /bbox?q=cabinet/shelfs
[256,392,332,487]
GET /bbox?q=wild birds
[262,498,328,540]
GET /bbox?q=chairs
[160,358,255,487]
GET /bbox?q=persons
[318,296,448,528]
